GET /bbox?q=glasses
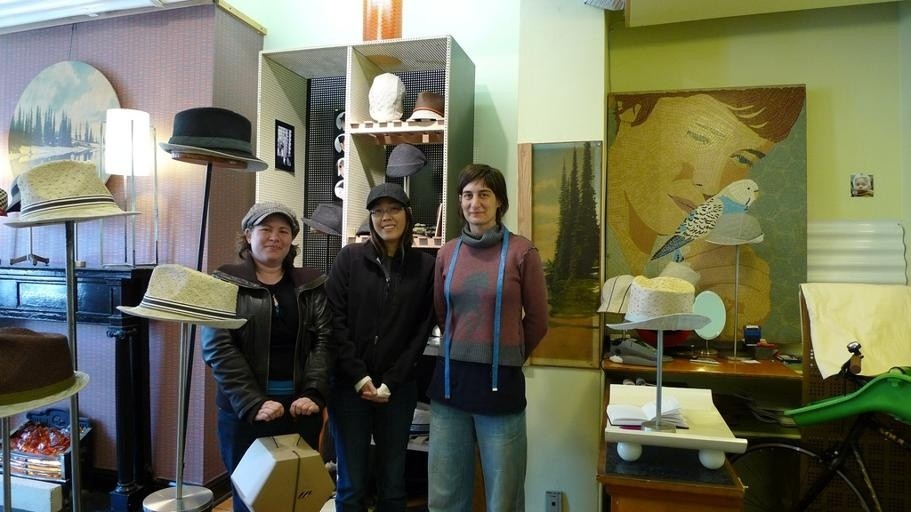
[369,207,401,216]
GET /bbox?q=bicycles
[725,341,911,511]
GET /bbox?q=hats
[241,201,300,240]
[386,143,427,178]
[301,203,342,236]
[366,183,410,207]
[0,160,143,229]
[413,92,444,117]
[368,72,406,122]
[596,273,712,331]
[0,326,90,419]
[159,107,268,172]
[356,218,370,235]
[115,263,248,331]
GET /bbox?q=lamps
[99,108,158,266]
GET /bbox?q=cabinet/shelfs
[255,35,475,451]
[596,351,803,511]
[0,1,268,488]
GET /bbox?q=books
[749,400,796,428]
[606,395,690,430]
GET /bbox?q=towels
[799,282,911,380]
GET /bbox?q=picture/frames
[275,119,294,172]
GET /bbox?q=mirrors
[693,290,727,358]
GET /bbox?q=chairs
[783,367,911,427]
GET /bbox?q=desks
[0,267,167,512]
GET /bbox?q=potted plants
[545,490,563,512]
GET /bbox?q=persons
[427,163,549,512]
[608,86,805,341]
[324,183,438,512]
[200,201,336,511]
[852,174,873,197]
